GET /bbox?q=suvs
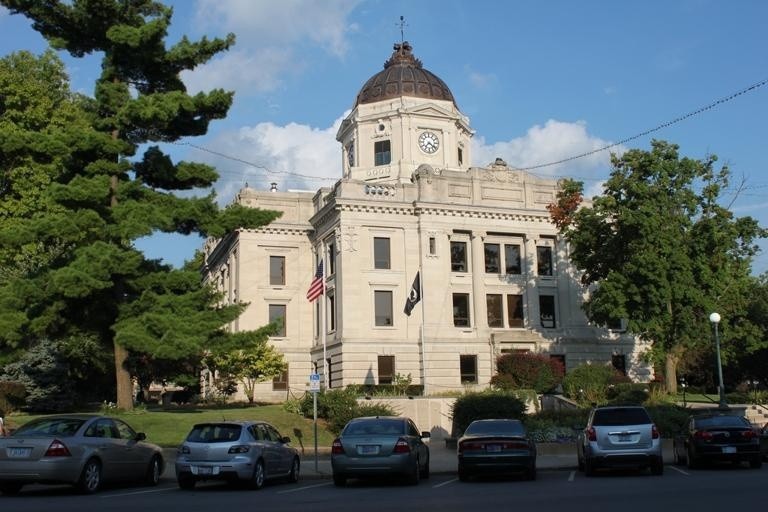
[573,403,665,476]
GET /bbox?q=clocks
[418,132,439,154]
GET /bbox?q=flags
[306,258,323,303]
[403,270,421,316]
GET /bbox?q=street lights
[709,309,731,412]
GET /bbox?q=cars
[0,411,169,496]
[670,413,765,471]
[329,413,432,486]
[173,419,304,491]
[757,420,768,464]
[455,417,538,483]
